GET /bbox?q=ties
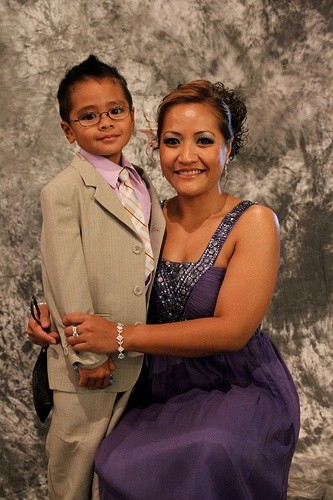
[118,169,155,281]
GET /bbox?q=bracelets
[72,326,79,338]
[115,322,126,360]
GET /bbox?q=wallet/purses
[30,296,53,422]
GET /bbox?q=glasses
[68,105,133,127]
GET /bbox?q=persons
[26,80,301,500]
[39,54,166,500]
